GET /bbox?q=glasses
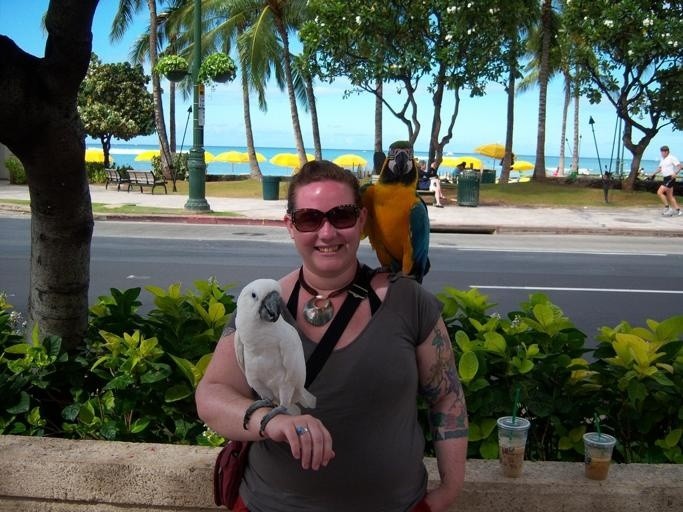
[284,203,366,233]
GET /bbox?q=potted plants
[154,55,189,82]
[197,53,236,89]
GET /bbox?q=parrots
[235,279,316,436]
[359,142,431,285]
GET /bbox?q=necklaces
[303,294,333,327]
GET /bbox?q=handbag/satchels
[212,440,252,506]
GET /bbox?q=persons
[648,145,683,217]
[193,159,470,512]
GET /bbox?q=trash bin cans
[262,176,281,200]
[481,169,496,184]
[456,168,481,207]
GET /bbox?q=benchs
[103,168,127,191]
[124,169,169,194]
[370,175,436,204]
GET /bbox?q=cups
[496,417,531,479]
[582,432,619,479]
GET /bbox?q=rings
[297,427,309,436]
